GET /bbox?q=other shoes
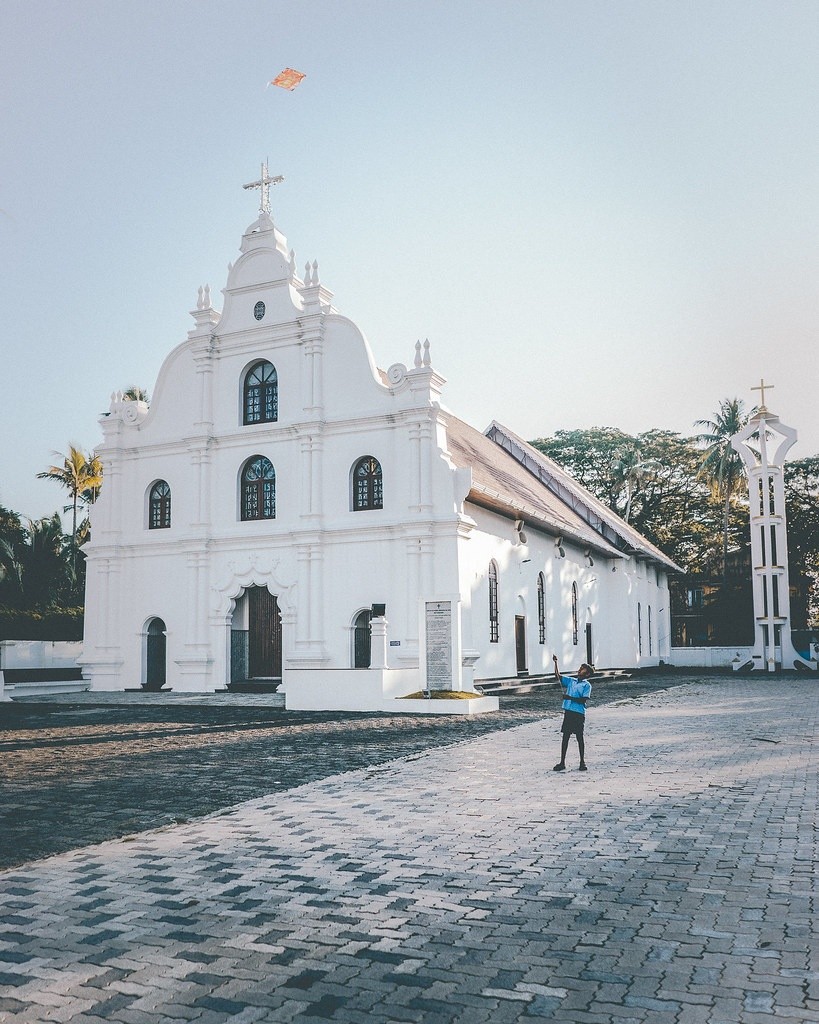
[553,762,565,771]
[580,762,588,770]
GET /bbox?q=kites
[264,68,306,92]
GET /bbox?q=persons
[553,654,595,771]
